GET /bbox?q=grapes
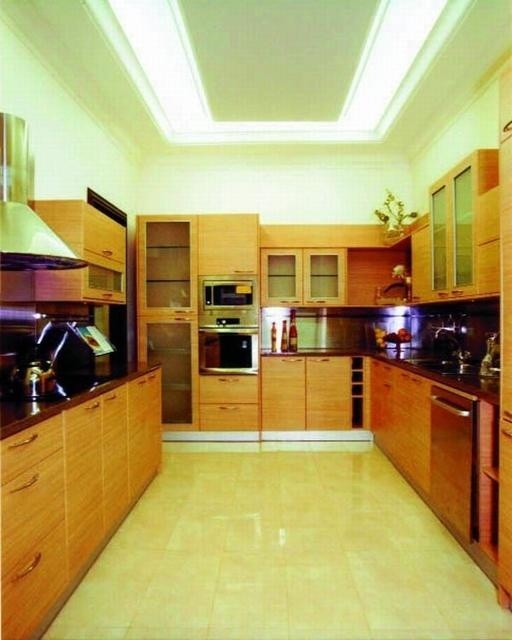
[383,333,398,342]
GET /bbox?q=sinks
[402,356,481,376]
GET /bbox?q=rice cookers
[374,307,423,349]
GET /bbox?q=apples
[397,328,411,341]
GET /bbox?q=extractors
[1,113,88,270]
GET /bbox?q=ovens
[427,386,477,547]
[198,327,259,374]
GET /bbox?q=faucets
[431,312,473,363]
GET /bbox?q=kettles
[11,361,57,401]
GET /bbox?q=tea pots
[478,336,500,377]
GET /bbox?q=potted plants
[373,188,419,245]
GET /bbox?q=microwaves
[203,280,256,309]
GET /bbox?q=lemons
[374,329,386,348]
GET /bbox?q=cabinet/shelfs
[493,64,512,613]
[473,184,500,298]
[134,212,200,443]
[29,198,129,307]
[64,379,130,608]
[0,405,72,640]
[198,212,261,276]
[260,356,373,442]
[422,147,498,303]
[124,362,164,512]
[395,369,431,500]
[365,361,395,461]
[408,225,435,306]
[347,235,412,310]
[261,247,349,310]
[197,374,261,442]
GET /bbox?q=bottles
[272,322,277,352]
[281,320,288,352]
[289,309,297,351]
